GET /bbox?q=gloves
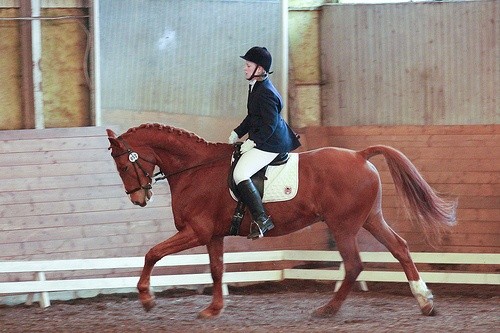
[240,139,257,154]
[229,130,238,144]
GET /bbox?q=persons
[229,45,301,238]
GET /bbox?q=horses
[106,122,460,318]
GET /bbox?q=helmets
[240,46,272,73]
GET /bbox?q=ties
[249,84,252,96]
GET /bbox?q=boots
[237,179,274,239]
